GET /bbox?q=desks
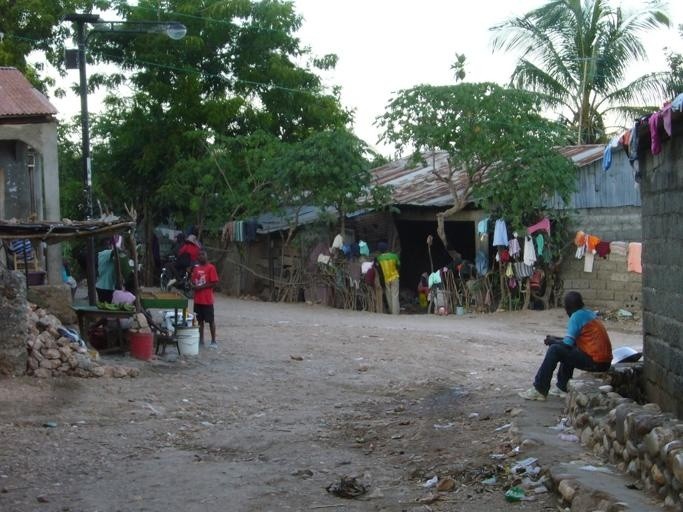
[141,292,188,333]
[67,300,136,357]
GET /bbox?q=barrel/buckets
[130,333,154,361]
[176,327,200,356]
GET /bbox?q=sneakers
[517,386,547,402]
[207,341,218,350]
[548,387,568,399]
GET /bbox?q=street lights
[62,14,187,307]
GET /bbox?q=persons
[518,290,613,401]
[192,248,219,348]
[166,234,200,289]
[418,271,429,307]
[374,241,401,314]
[89,238,117,341]
[107,277,137,329]
[61,256,77,302]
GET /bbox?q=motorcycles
[158,255,194,300]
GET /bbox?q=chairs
[3,239,39,274]
